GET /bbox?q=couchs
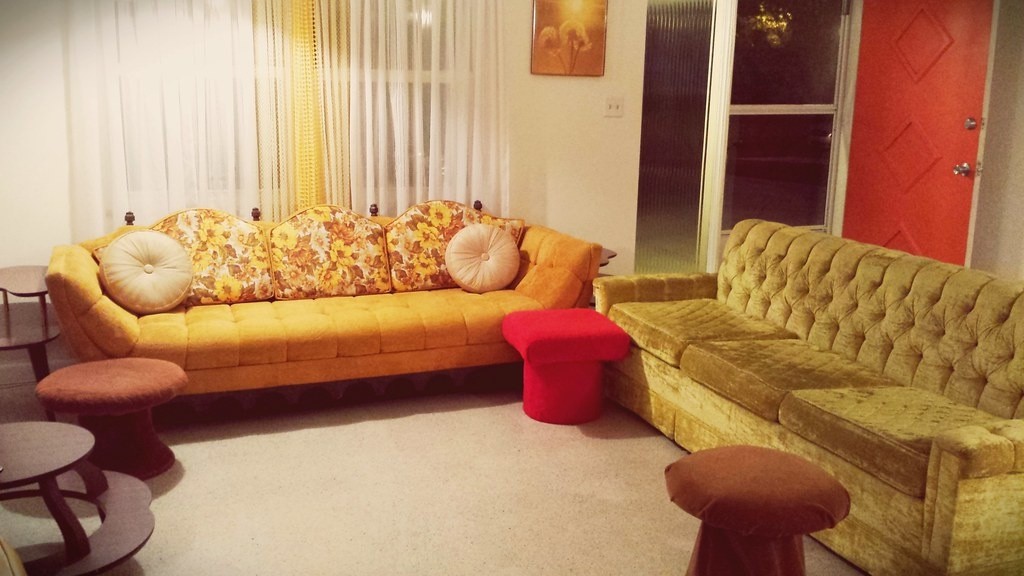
[592,216,1024,576]
[53,202,617,394]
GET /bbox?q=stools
[663,445,851,576]
[501,309,629,425]
[34,359,187,480]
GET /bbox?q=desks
[0,419,155,576]
[0,265,50,339]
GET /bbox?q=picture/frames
[530,0,608,77]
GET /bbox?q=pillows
[268,204,392,300]
[100,231,193,313]
[445,223,520,292]
[93,209,275,306]
[385,200,524,291]
